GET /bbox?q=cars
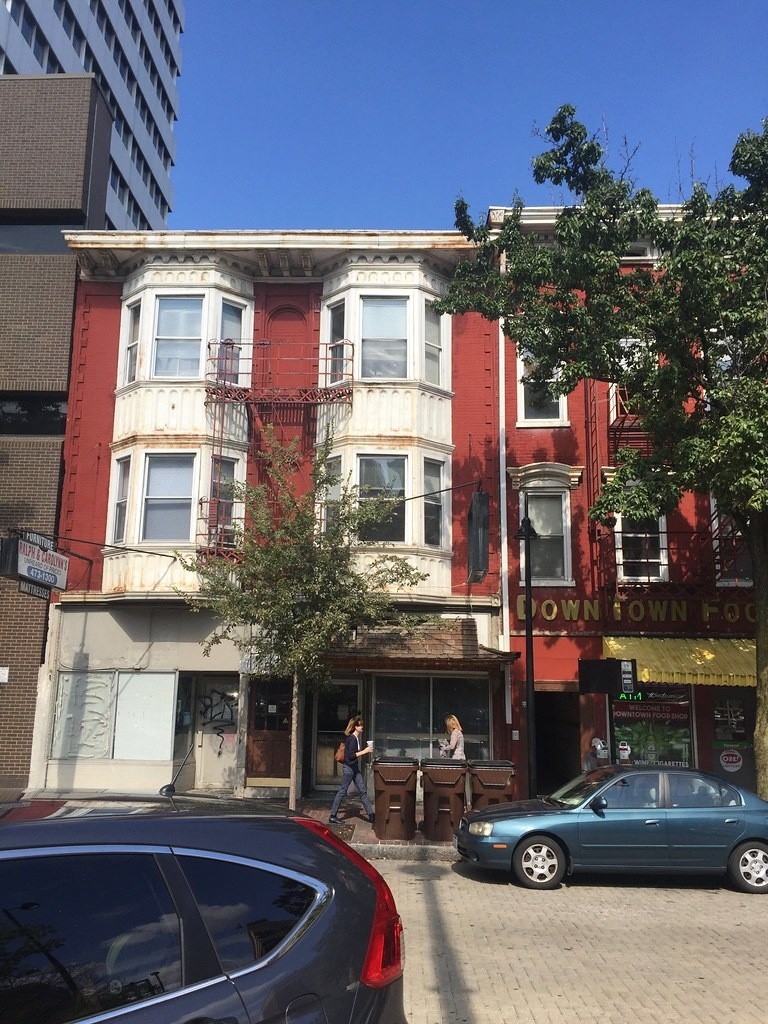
[450,764,768,893]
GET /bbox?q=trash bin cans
[418,757,467,842]
[467,759,516,810]
[371,756,420,841]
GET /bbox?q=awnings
[602,636,757,687]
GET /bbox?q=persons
[582,737,611,773]
[439,713,466,760]
[329,715,376,824]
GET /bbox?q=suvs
[0,781,407,1023]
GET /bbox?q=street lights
[514,516,541,799]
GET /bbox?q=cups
[366,741,374,753]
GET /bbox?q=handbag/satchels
[334,735,354,764]
[438,738,450,758]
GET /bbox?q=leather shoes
[329,817,345,824]
[369,815,375,823]
